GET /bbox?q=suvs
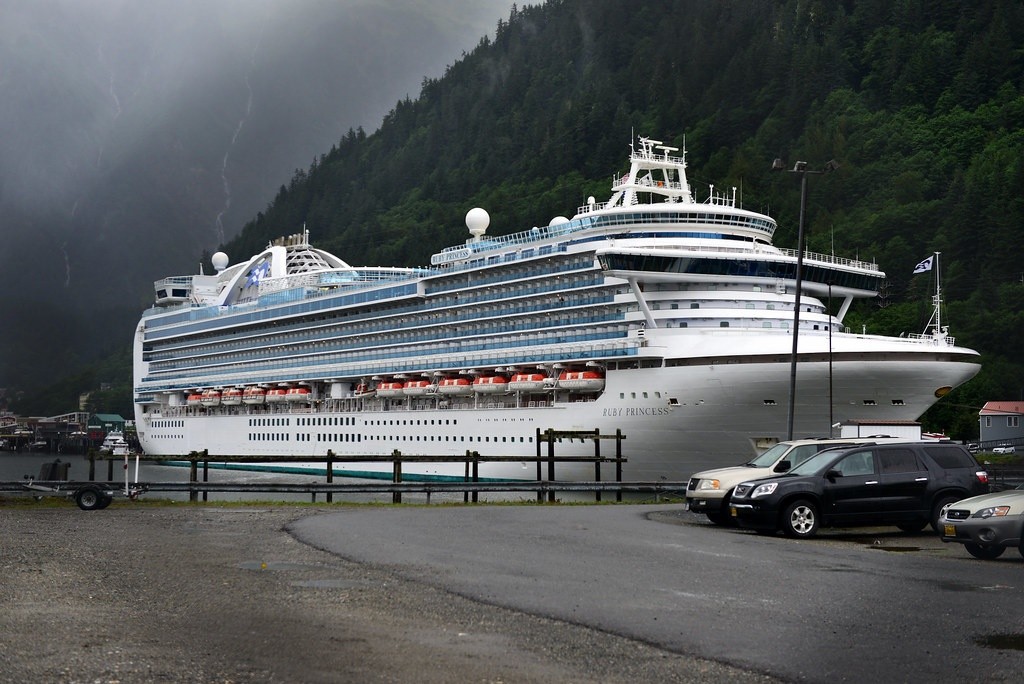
[727,440,990,539]
[684,435,902,527]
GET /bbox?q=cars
[967,443,981,455]
[937,482,1024,560]
[993,444,1015,455]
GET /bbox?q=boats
[244,386,265,405]
[201,389,220,407]
[438,379,473,397]
[920,429,951,442]
[377,382,404,400]
[402,379,433,398]
[472,376,511,396]
[286,388,311,402]
[187,394,200,406]
[508,373,550,394]
[557,371,606,393]
[265,389,286,402]
[222,387,242,406]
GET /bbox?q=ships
[131,127,983,495]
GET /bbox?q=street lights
[770,158,840,444]
